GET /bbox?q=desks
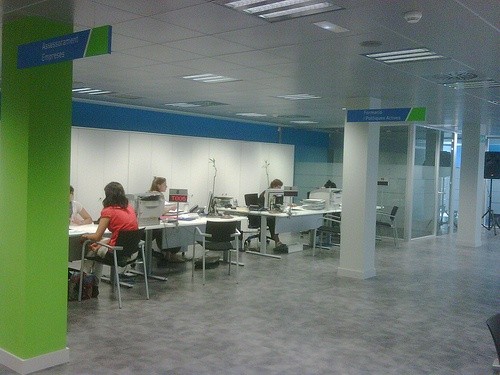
[128,211,253,282]
[319,203,385,245]
[213,204,261,218]
[246,203,330,258]
[68,221,134,289]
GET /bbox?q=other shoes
[275,242,282,247]
[167,255,187,263]
[92,285,99,298]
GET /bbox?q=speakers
[484,151,500,179]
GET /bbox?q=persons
[150,177,186,263]
[67,186,92,279]
[259,179,286,251]
[81,182,138,297]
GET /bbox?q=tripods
[481,180,500,236]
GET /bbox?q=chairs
[376,205,400,247]
[311,215,344,256]
[79,225,151,309]
[191,218,248,287]
[246,193,273,244]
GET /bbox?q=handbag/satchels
[69,272,99,300]
[195,257,219,269]
